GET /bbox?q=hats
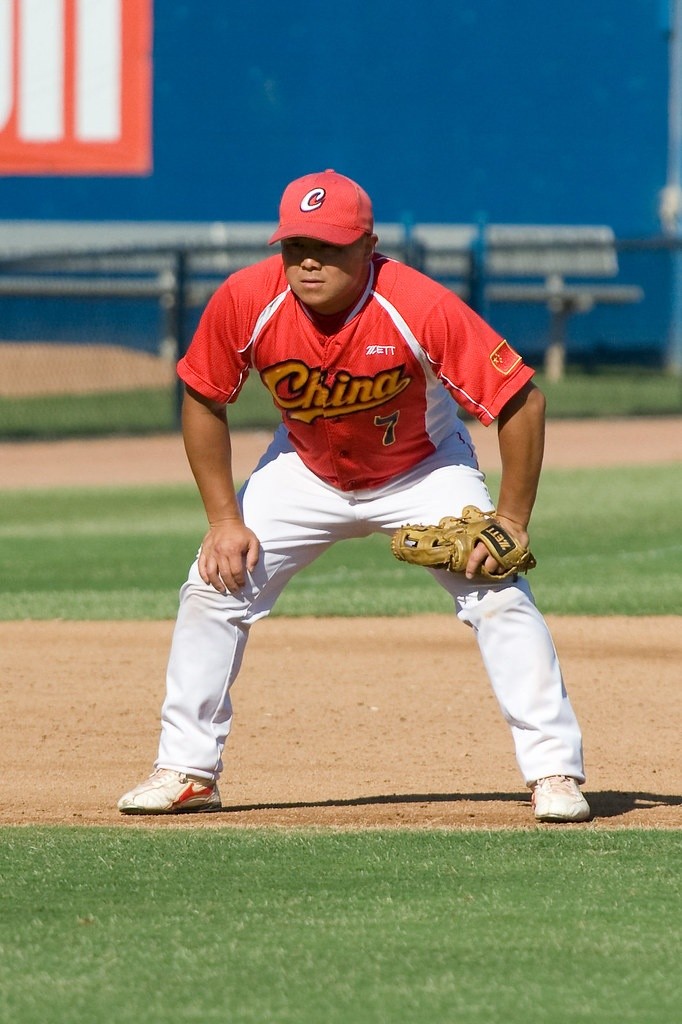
[268,169,373,247]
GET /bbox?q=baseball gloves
[391,506,537,582]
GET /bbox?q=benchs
[0,219,644,399]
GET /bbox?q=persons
[119,173,592,820]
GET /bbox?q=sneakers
[117,768,221,815]
[531,775,590,822]
